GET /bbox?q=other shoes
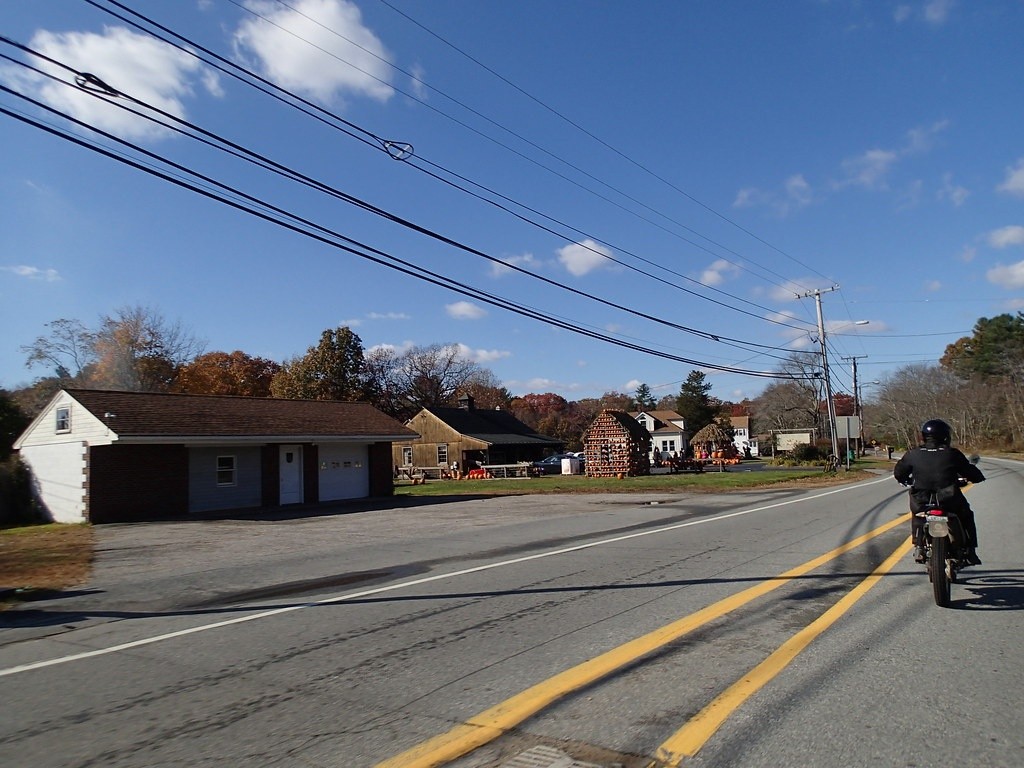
[967,551,982,565]
[914,548,927,564]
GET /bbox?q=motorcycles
[897,453,985,607]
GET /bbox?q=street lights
[819,320,870,467]
[858,380,879,457]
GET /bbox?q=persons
[680,448,687,470]
[895,419,984,564]
[654,449,662,467]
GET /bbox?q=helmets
[922,419,951,447]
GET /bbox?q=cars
[534,452,586,475]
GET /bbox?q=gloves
[902,477,912,486]
